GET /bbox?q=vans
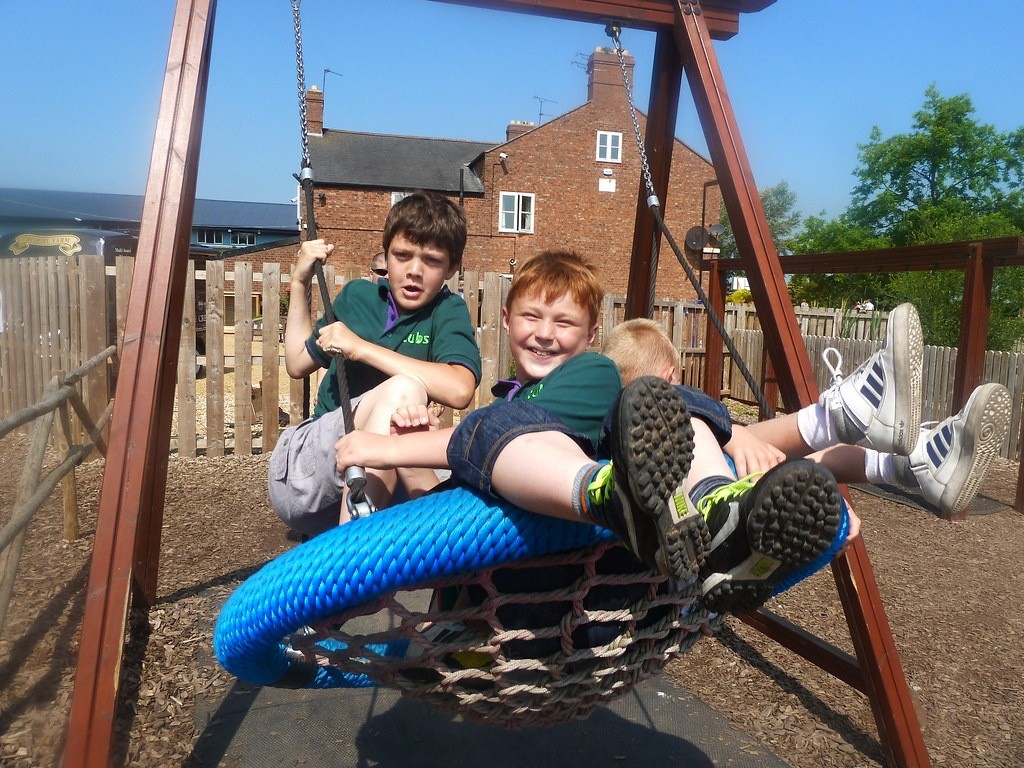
[0,225,220,382]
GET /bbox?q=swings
[213,0,849,734]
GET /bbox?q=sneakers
[893,382,1013,512]
[819,303,924,456]
[398,618,511,684]
[586,376,713,580]
[699,458,841,616]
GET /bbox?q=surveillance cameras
[500,153,508,159]
[291,198,297,203]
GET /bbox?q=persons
[267,191,1013,692]
